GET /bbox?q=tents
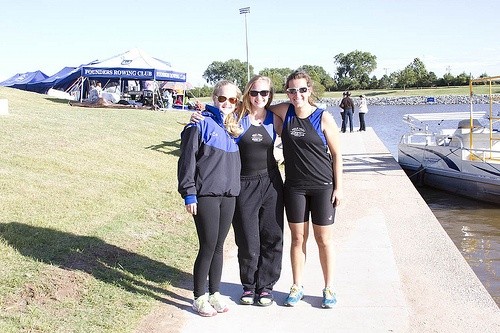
[0,59,100,101]
[81,50,187,110]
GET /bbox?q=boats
[397,74,500,205]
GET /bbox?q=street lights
[238,6,251,83]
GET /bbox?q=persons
[339,91,355,133]
[96,81,102,93]
[267,71,341,308]
[178,80,241,316]
[190,77,285,306]
[357,95,368,131]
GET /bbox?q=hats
[343,92,347,95]
[347,92,351,95]
[359,95,363,97]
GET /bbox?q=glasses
[249,90,270,96]
[214,94,239,104]
[287,88,310,93]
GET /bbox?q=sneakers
[192,294,218,317]
[207,292,228,313]
[257,291,274,306]
[320,288,336,307]
[240,290,255,305]
[284,284,304,307]
